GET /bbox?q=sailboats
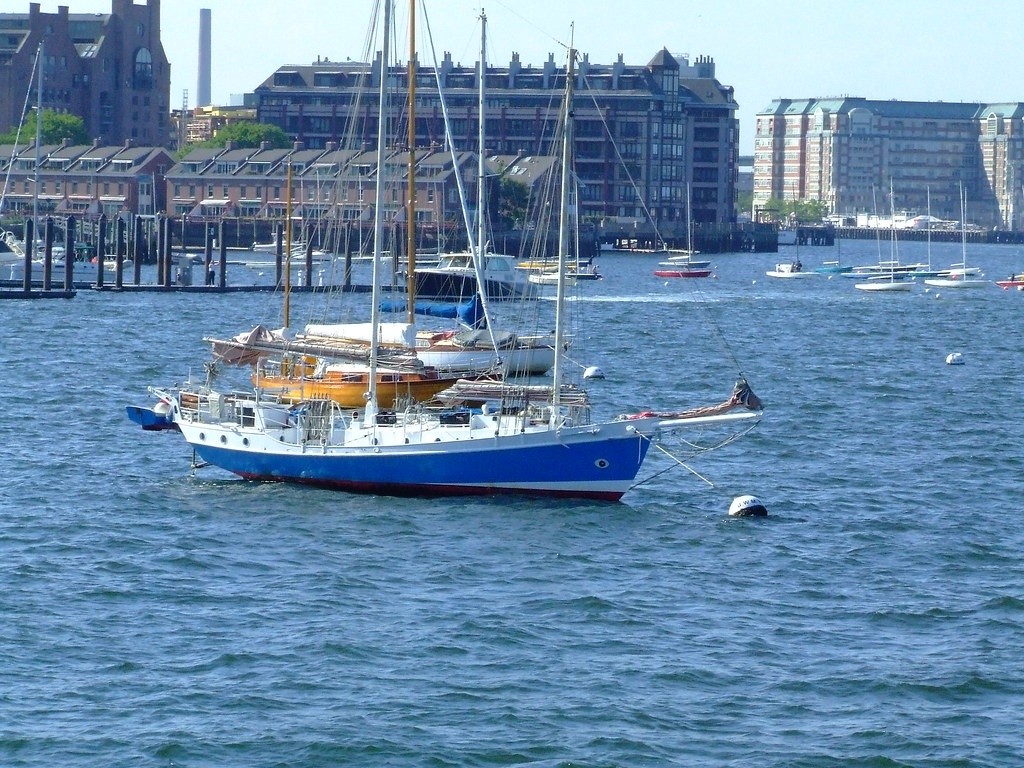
[0,1,1024,505]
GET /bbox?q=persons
[791,261,802,272]
[208,260,220,285]
